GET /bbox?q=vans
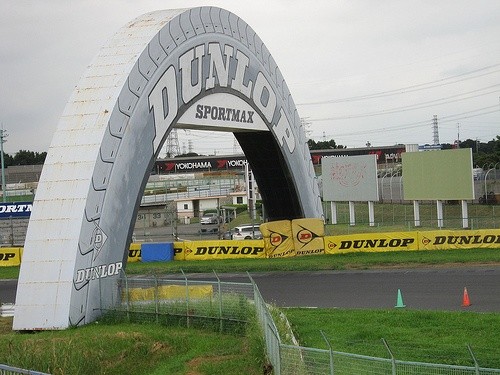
[473,168,484,181]
[233,226,263,240]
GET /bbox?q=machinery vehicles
[479,191,500,206]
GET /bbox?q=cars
[201,213,222,225]
[220,230,233,240]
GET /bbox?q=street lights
[0,129,10,203]
